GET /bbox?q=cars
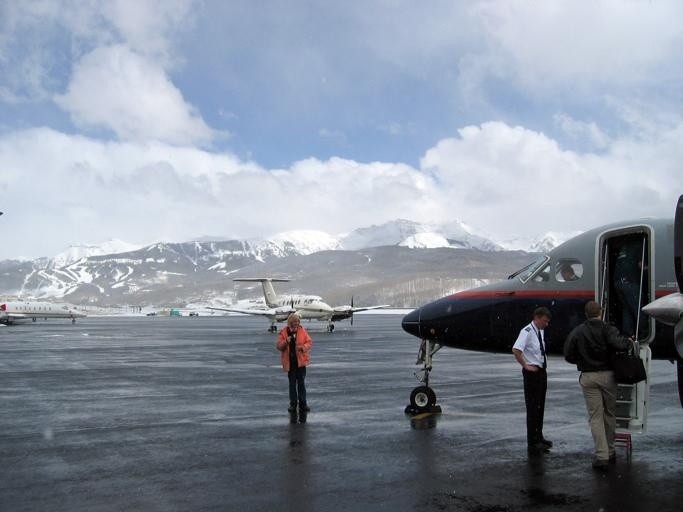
[189,311,199,316]
[146,311,158,316]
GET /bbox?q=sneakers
[288,404,310,411]
[592,449,616,469]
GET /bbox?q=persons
[275,313,313,411]
[558,263,581,281]
[562,300,629,467]
[512,306,553,451]
[612,234,649,341]
[286,407,312,424]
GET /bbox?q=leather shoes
[527,438,552,451]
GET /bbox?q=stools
[614,428,632,458]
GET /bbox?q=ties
[538,330,544,356]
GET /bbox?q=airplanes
[397,194,682,433]
[1,298,90,326]
[203,276,394,334]
